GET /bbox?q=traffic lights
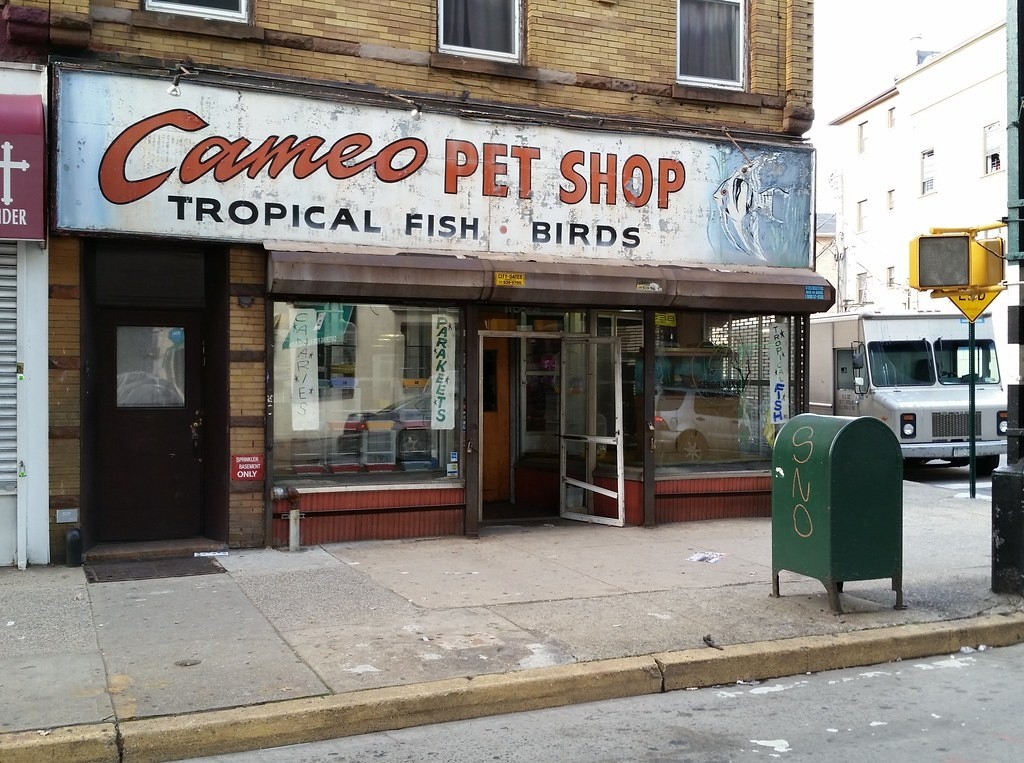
[910,233,972,290]
[972,237,1006,284]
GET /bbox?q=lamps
[168,62,195,97]
[723,126,753,174]
[389,92,423,121]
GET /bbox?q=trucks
[713,310,1012,478]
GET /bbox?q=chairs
[914,359,942,382]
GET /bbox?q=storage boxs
[512,453,572,512]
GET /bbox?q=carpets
[81,555,228,584]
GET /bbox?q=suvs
[652,387,740,462]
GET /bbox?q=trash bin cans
[771,413,905,613]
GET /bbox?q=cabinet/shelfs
[512,317,643,505]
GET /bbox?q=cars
[336,393,459,462]
